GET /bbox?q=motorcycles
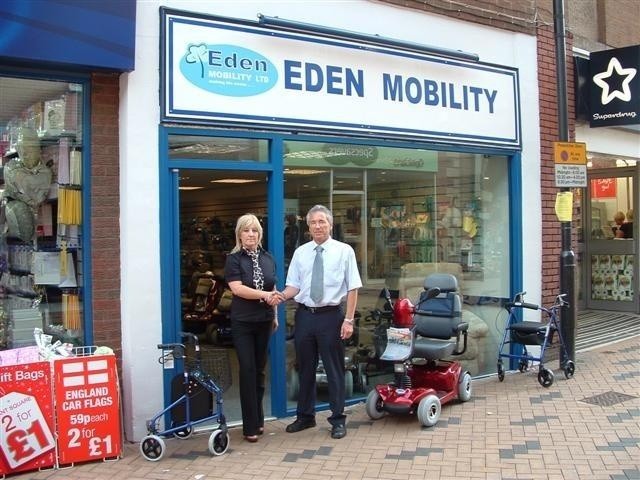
[365,274,473,428]
[289,311,370,400]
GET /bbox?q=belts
[295,301,340,315]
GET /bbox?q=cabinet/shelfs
[0,135,73,348]
[0,345,123,480]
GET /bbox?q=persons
[222,211,287,442]
[615,208,634,238]
[612,210,625,234]
[264,203,365,438]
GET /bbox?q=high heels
[256,426,264,434]
[244,434,259,443]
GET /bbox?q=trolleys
[498,292,576,388]
[139,332,233,463]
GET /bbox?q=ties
[242,246,266,303]
[310,245,323,302]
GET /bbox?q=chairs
[398,276,487,378]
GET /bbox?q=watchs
[342,318,356,326]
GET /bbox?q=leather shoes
[286,419,317,433]
[331,423,346,439]
